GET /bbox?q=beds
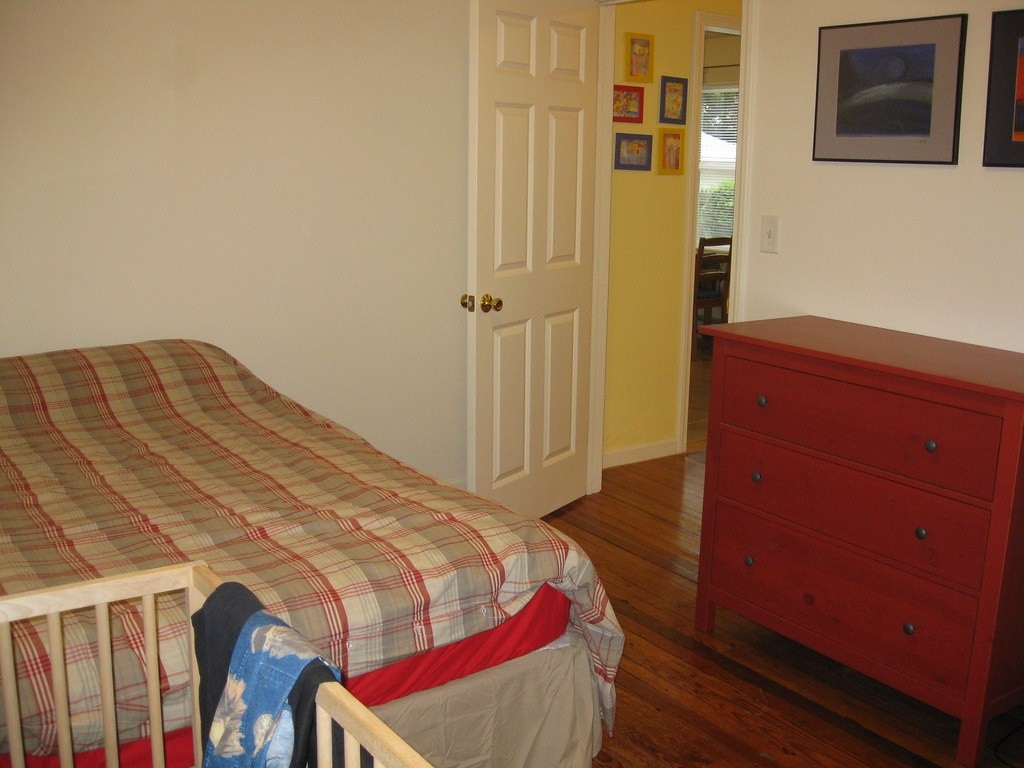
[1,560,432,768]
[0,339,626,768]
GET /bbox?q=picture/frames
[982,9,1024,168]
[812,13,969,166]
[659,76,689,125]
[625,32,655,83]
[615,133,653,171]
[657,128,685,174]
[613,85,644,123]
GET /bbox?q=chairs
[692,236,732,360]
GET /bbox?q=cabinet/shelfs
[693,314,1024,768]
